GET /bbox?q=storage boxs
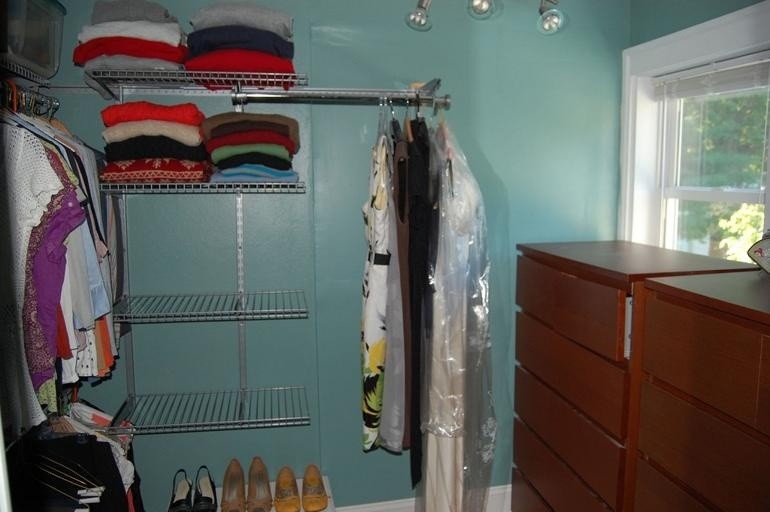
[1,0,67,79]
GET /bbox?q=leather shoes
[221,458,247,512]
[302,463,328,512]
[274,466,302,512]
[247,456,273,512]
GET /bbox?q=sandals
[167,468,193,512]
[193,465,218,512]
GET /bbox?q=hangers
[25,404,136,512]
[370,87,452,158]
[3,79,63,125]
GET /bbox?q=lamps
[536,0,567,36]
[464,1,494,15]
[406,0,436,32]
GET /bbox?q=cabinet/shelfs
[508,237,764,512]
[622,267,769,511]
[84,66,310,437]
